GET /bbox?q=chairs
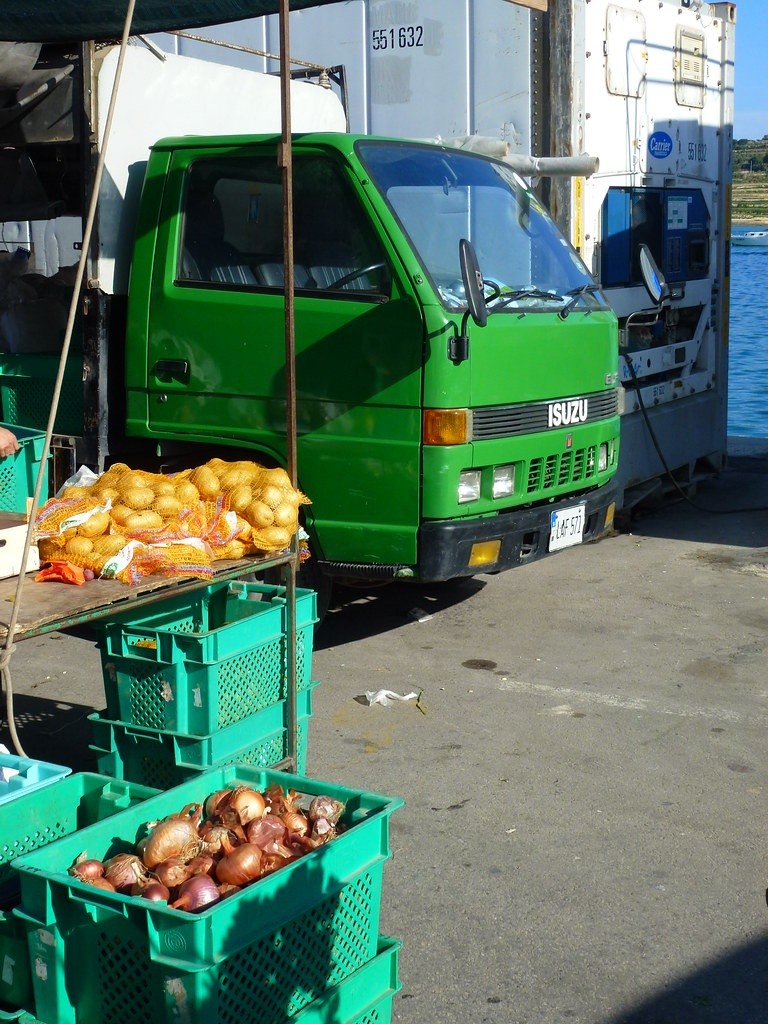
[181,195,259,287]
[255,254,313,290]
[311,197,370,290]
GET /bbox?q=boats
[732,231,768,247]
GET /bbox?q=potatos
[36,459,299,578]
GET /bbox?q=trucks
[0,43,623,634]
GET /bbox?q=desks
[0,511,299,778]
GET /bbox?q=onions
[67,784,343,914]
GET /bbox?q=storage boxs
[95,580,321,736]
[0,752,407,1024]
[0,351,84,514]
[88,679,322,792]
